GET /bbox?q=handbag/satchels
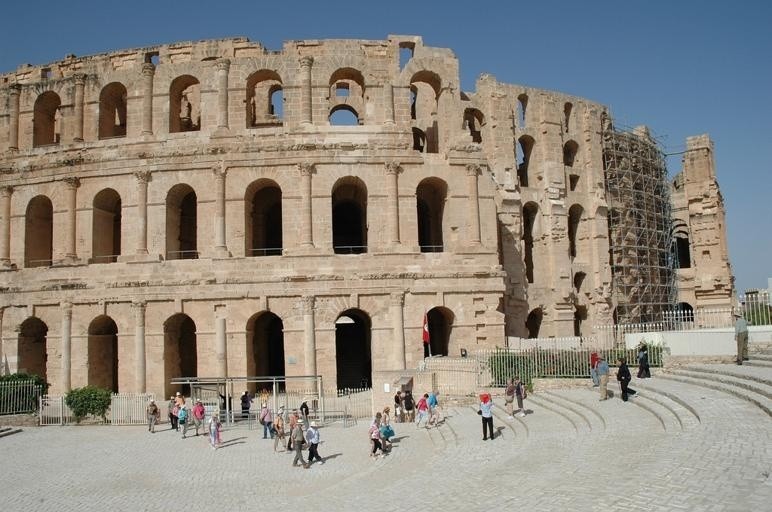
[503,396,514,403]
[179,420,186,425]
[521,393,529,400]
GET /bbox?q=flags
[422,311,430,344]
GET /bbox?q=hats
[149,390,221,418]
[374,408,391,424]
[732,311,744,318]
[479,393,490,403]
[594,353,606,360]
[262,397,323,429]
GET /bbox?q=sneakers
[367,448,393,462]
[261,446,326,471]
[506,413,527,421]
[599,391,640,405]
[148,427,224,450]
[635,376,653,381]
[414,423,443,431]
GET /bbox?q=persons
[259,387,324,469]
[167,392,232,449]
[479,394,495,440]
[590,350,599,386]
[615,358,639,403]
[241,391,254,418]
[733,312,750,365]
[635,341,651,380]
[506,379,515,420]
[597,357,609,401]
[361,376,369,389]
[369,390,441,459]
[145,400,158,433]
[513,376,525,417]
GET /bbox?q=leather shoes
[481,436,495,441]
